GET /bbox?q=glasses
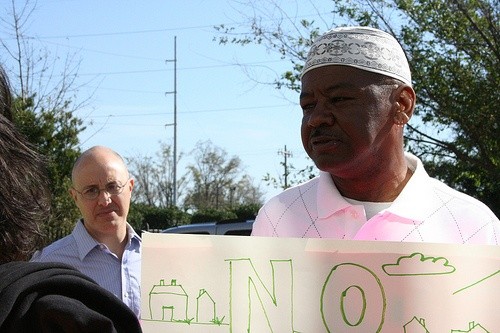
[72,182,128,200]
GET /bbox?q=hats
[300,27,411,85]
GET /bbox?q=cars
[163,219,255,235]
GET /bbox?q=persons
[0,64,142,333]
[27,144,142,325]
[249,25,500,333]
